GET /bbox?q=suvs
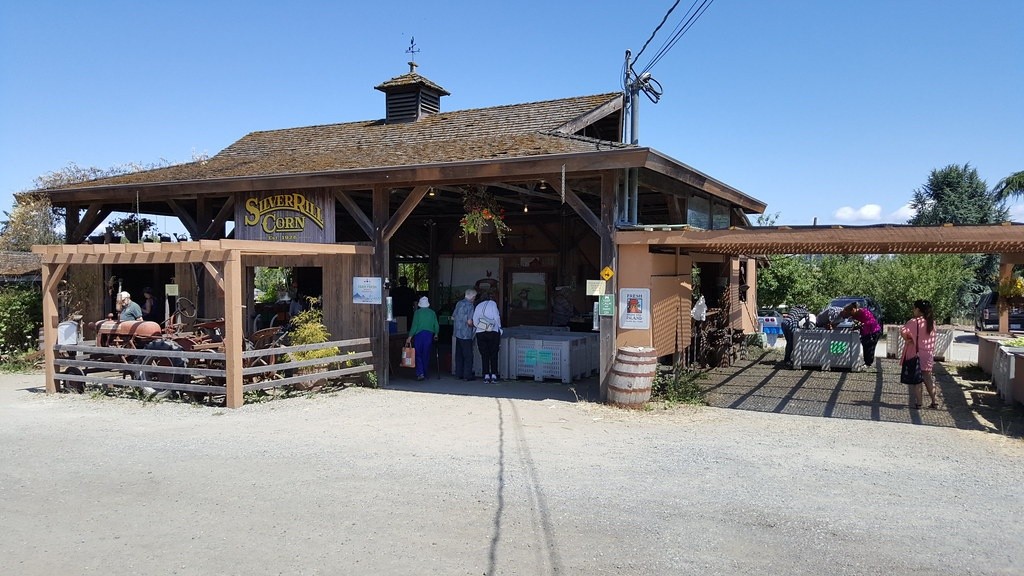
[827,296,883,336]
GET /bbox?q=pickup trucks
[973,292,1024,341]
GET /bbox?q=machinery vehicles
[62,297,255,401]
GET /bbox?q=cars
[757,309,786,338]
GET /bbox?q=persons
[451,288,477,381]
[120,291,144,321]
[846,306,881,369]
[473,291,502,384]
[781,306,810,364]
[405,296,439,381]
[141,287,160,326]
[899,299,940,411]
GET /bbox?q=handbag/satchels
[901,355,924,385]
[399,342,415,368]
[476,315,495,331]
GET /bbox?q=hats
[418,297,430,307]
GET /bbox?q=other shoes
[491,374,502,384]
[418,374,425,381]
[862,364,872,369]
[464,377,476,381]
[483,374,490,383]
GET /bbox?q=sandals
[912,403,921,409]
[930,403,939,408]
